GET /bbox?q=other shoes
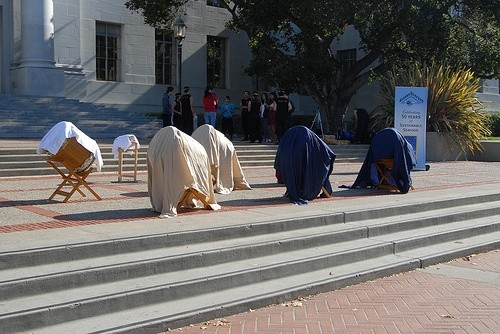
[257,140,262,143]
[250,140,255,143]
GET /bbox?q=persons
[161,86,296,144]
[353,107,370,144]
